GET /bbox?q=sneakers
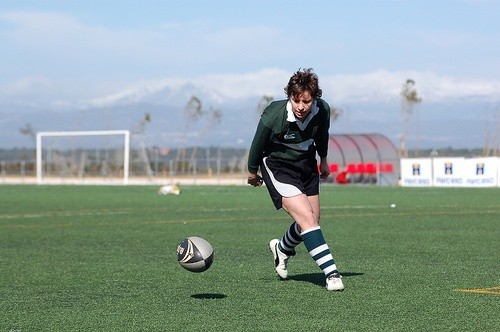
[325,278,344,291]
[269,239,287,279]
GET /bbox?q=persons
[247,68,344,292]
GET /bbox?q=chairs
[317,163,398,187]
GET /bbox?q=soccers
[176,236,215,273]
